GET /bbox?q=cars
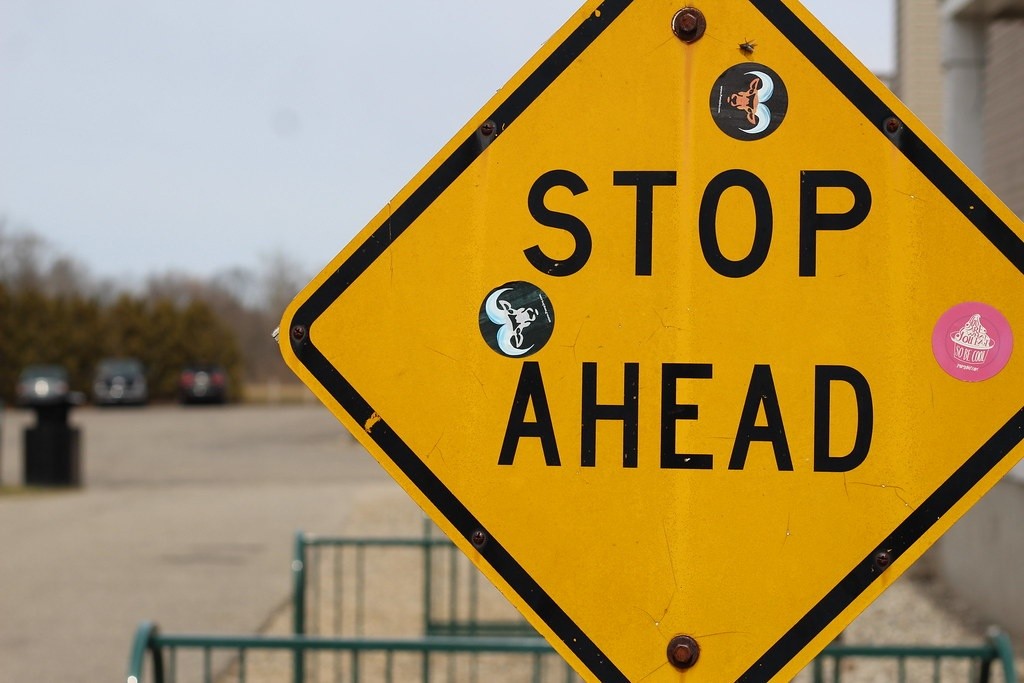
[16,361,70,406]
[92,358,148,407]
[181,365,225,403]
